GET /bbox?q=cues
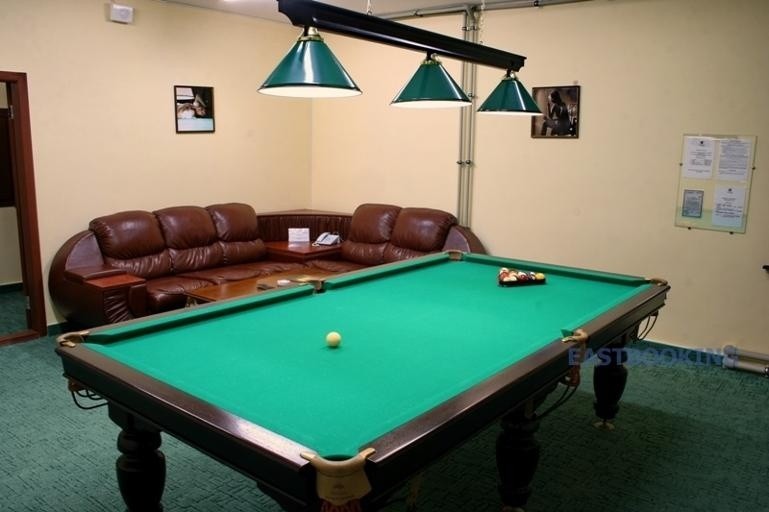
[722,344,769,377]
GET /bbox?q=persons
[178,93,212,120]
[541,92,571,137]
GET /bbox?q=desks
[54,249,671,512]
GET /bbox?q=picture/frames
[530,86,581,139]
[174,85,215,133]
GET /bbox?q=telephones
[312,232,340,247]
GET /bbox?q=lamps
[255,0,544,118]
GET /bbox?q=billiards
[499,266,545,285]
[327,331,341,347]
